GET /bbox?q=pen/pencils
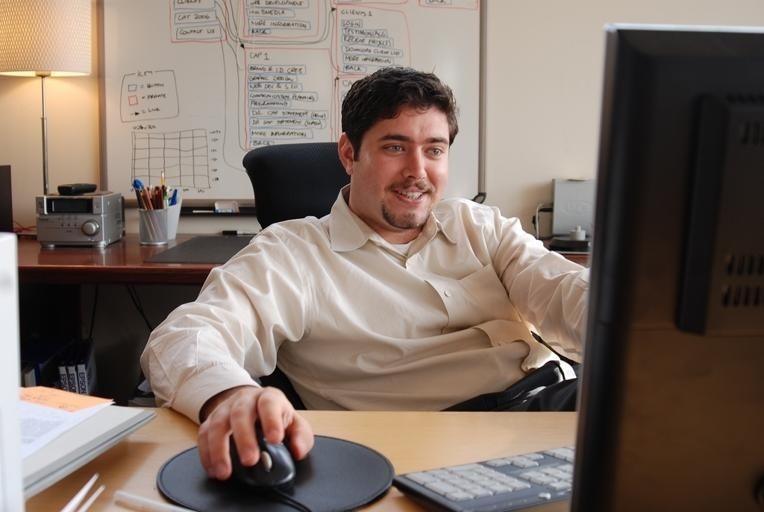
[222,231,260,234]
[133,171,178,242]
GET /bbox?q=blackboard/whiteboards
[98,0,487,212]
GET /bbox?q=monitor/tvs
[574,24,763,512]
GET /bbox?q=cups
[569,229,587,241]
[167,200,183,241]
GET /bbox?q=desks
[13,230,258,286]
[25,407,578,512]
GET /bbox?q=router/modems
[550,175,595,235]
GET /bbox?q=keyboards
[392,445,574,512]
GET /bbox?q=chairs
[242,142,350,411]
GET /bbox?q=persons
[139,67,592,481]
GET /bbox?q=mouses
[226,433,297,493]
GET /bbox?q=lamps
[0,1,93,238]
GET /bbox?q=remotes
[58,183,97,195]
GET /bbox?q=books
[0,385,157,511]
[0,231,29,511]
